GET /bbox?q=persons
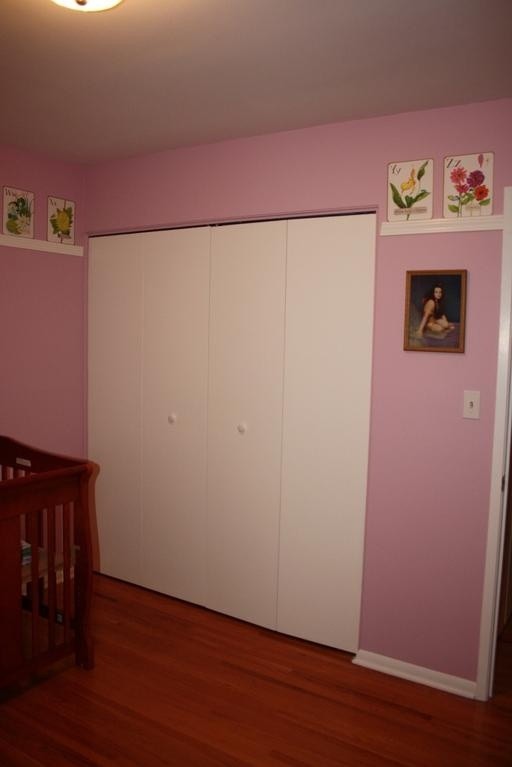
[411,282,455,339]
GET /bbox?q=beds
[0,434,93,699]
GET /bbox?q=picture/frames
[403,269,467,353]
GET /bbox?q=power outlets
[463,391,480,419]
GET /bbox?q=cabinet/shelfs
[87,214,377,655]
